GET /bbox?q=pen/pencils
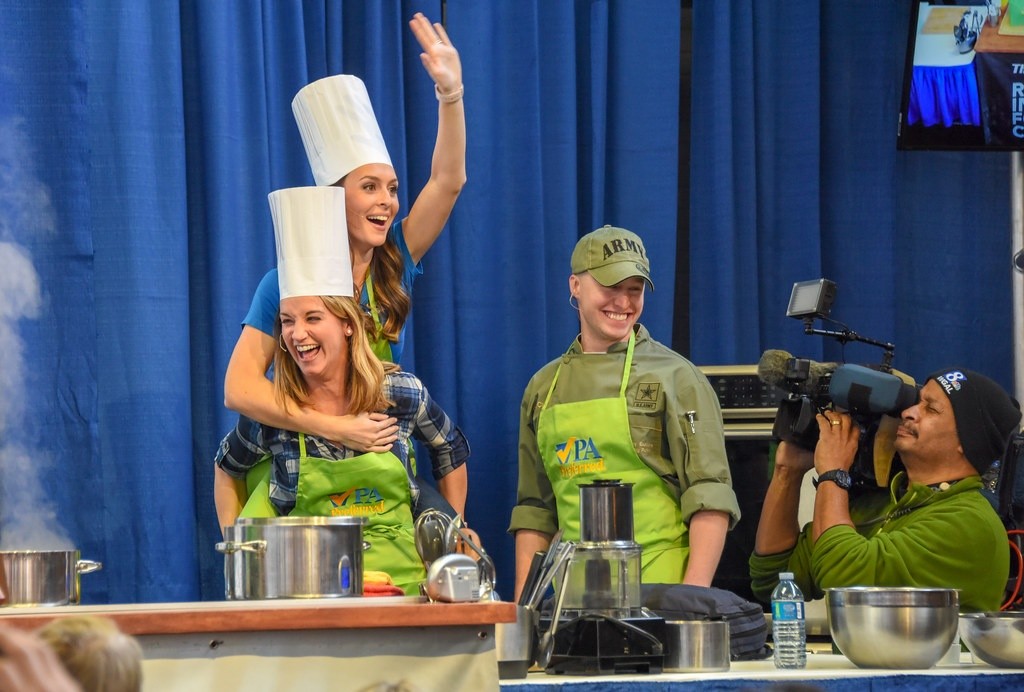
[686,411,696,434]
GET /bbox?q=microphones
[757,348,838,396]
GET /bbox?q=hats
[267,187,354,301]
[570,224,654,293]
[924,367,1022,476]
[290,75,393,187]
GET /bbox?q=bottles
[771,573,806,668]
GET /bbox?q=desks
[0,594,518,692]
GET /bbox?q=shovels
[457,528,481,560]
[527,528,564,607]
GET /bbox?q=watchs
[812,468,852,491]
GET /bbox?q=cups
[665,615,731,672]
[495,604,534,680]
[987,8,1000,28]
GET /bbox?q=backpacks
[538,582,773,661]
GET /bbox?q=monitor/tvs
[896,0,1024,152]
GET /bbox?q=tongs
[528,541,576,669]
[518,551,547,667]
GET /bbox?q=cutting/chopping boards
[998,4,1024,36]
[920,7,970,34]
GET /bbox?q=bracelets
[434,84,463,102]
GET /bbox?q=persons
[223,13,467,531]
[0,616,142,692]
[506,225,742,604]
[213,186,472,597]
[748,367,1023,653]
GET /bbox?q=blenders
[539,479,672,675]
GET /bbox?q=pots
[0,551,101,609]
[215,517,372,600]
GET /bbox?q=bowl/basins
[958,611,1023,668]
[826,586,962,671]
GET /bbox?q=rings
[832,420,840,425]
